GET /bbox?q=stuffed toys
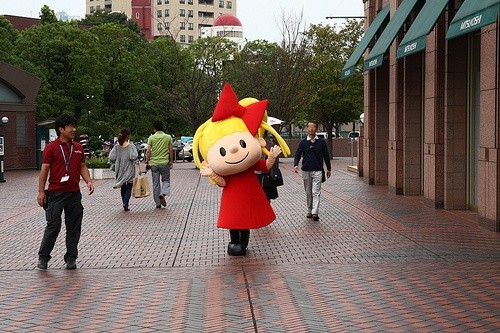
[192,83,291,256]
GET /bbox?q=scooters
[78,134,193,163]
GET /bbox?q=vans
[348,131,360,139]
[315,131,343,139]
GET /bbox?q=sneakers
[156,205,161,208]
[123,204,130,211]
[67,261,76,269]
[38,259,47,270]
[306,212,312,218]
[312,214,320,220]
[159,195,166,207]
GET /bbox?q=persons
[145,121,173,208]
[293,121,332,221]
[254,130,281,204]
[108,128,142,212]
[37,114,95,270]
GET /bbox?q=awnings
[446,0,500,41]
[340,3,390,80]
[361,0,417,72]
[396,0,450,59]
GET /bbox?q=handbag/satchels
[322,169,326,183]
[132,176,151,198]
[265,167,284,187]
